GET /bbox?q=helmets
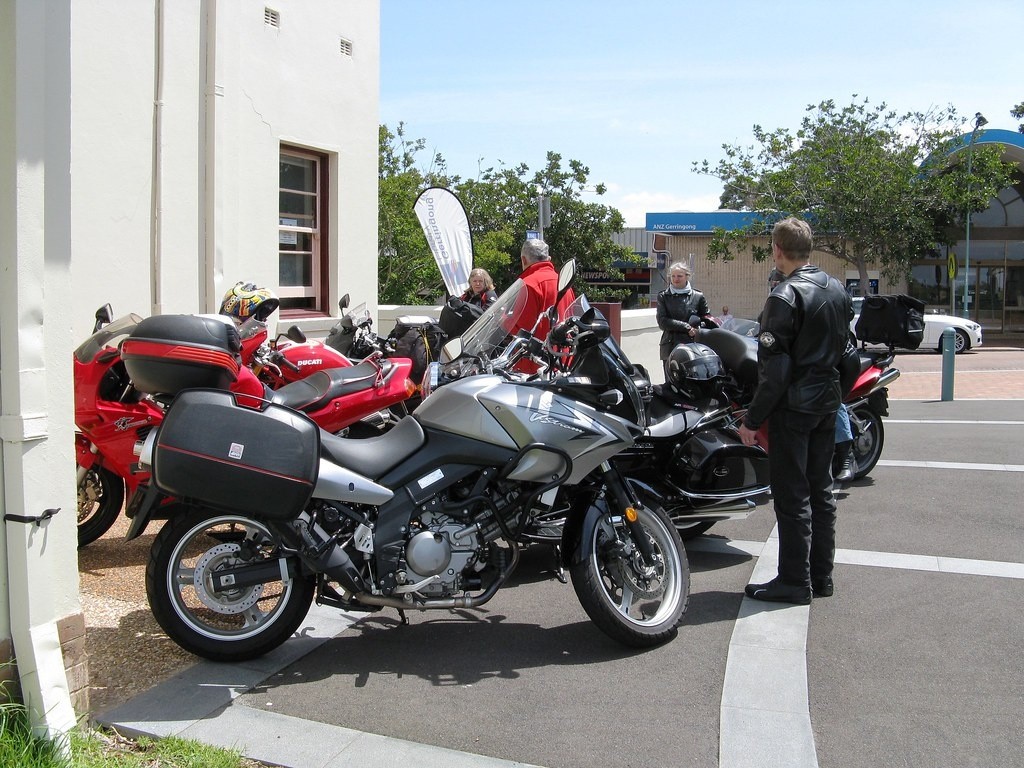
[218,282,280,327]
[665,342,727,402]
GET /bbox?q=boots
[836,441,859,483]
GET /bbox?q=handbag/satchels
[438,295,485,340]
[855,294,925,350]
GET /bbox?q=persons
[656,263,711,382]
[501,240,577,375]
[739,216,853,605]
[460,268,499,315]
[769,267,859,481]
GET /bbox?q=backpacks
[393,322,449,375]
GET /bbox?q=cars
[848,295,982,355]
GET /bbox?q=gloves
[768,266,786,296]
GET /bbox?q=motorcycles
[74,301,418,548]
[236,298,356,390]
[419,259,773,542]
[321,292,451,387]
[117,258,693,667]
[656,309,902,485]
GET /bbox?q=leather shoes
[811,576,833,596]
[745,575,812,604]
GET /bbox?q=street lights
[963,112,989,320]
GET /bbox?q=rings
[746,438,750,442]
